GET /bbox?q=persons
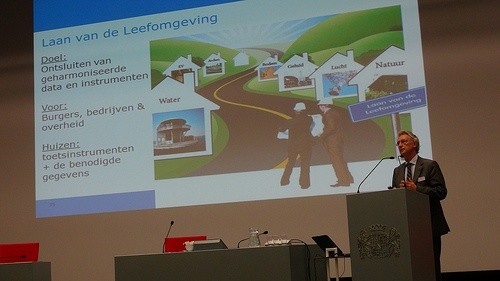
[392,129,451,281]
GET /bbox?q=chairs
[164,236,206,253]
[0,242,40,264]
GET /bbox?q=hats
[316,97,333,106]
[292,103,306,111]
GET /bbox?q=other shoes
[281,180,290,186]
[301,184,310,189]
[330,179,354,187]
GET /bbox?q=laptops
[312,234,351,258]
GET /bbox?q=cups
[186,242,194,251]
[267,235,273,246]
[273,235,280,246]
[248,228,260,247]
[281,234,287,245]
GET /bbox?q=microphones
[0,255,27,259]
[238,231,269,248]
[398,154,407,189]
[163,221,175,253]
[358,157,395,193]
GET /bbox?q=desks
[315,256,352,281]
[0,261,52,281]
[114,243,326,281]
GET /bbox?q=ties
[406,163,412,182]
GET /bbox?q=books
[194,238,228,251]
[268,239,290,244]
